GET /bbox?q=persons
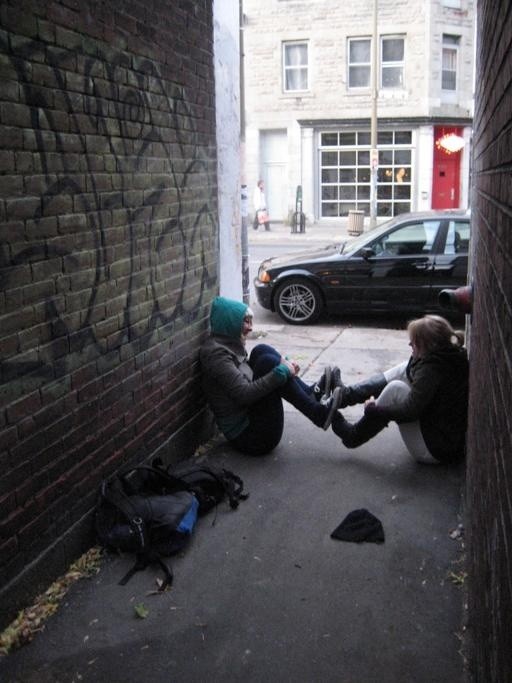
[331,312,468,465]
[199,297,343,459]
[252,179,271,231]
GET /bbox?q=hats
[244,308,254,318]
[331,509,384,543]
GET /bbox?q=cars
[253,207,471,326]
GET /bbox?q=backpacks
[96,466,198,556]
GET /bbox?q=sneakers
[322,386,341,431]
[313,366,331,400]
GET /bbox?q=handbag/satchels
[171,467,227,517]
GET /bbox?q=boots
[333,408,389,448]
[332,366,387,408]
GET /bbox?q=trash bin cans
[347,210,364,236]
